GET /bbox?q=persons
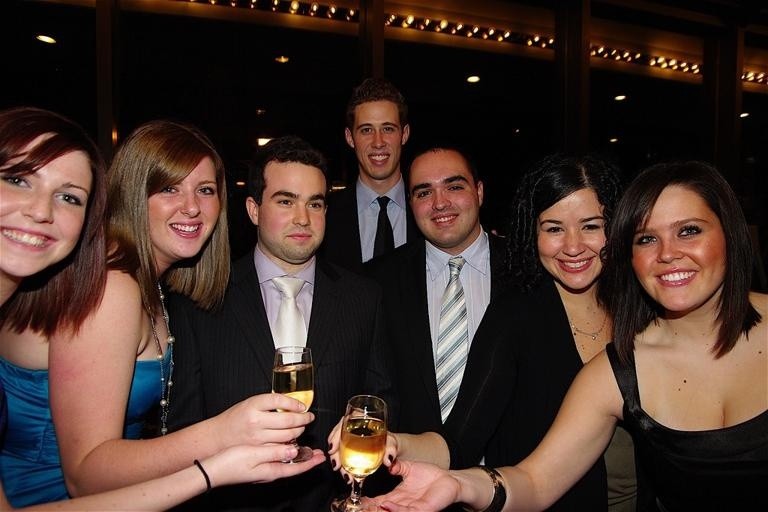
[325,140,636,511]
[348,134,527,511]
[166,133,401,511]
[357,155,767,510]
[320,71,424,281]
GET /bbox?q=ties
[435,256,468,425]
[271,277,308,366]
[373,196,394,259]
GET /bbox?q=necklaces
[566,302,607,341]
[145,275,175,438]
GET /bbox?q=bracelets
[460,456,507,512]
[193,460,211,494]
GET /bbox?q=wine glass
[271,346,316,466]
[329,394,389,512]
